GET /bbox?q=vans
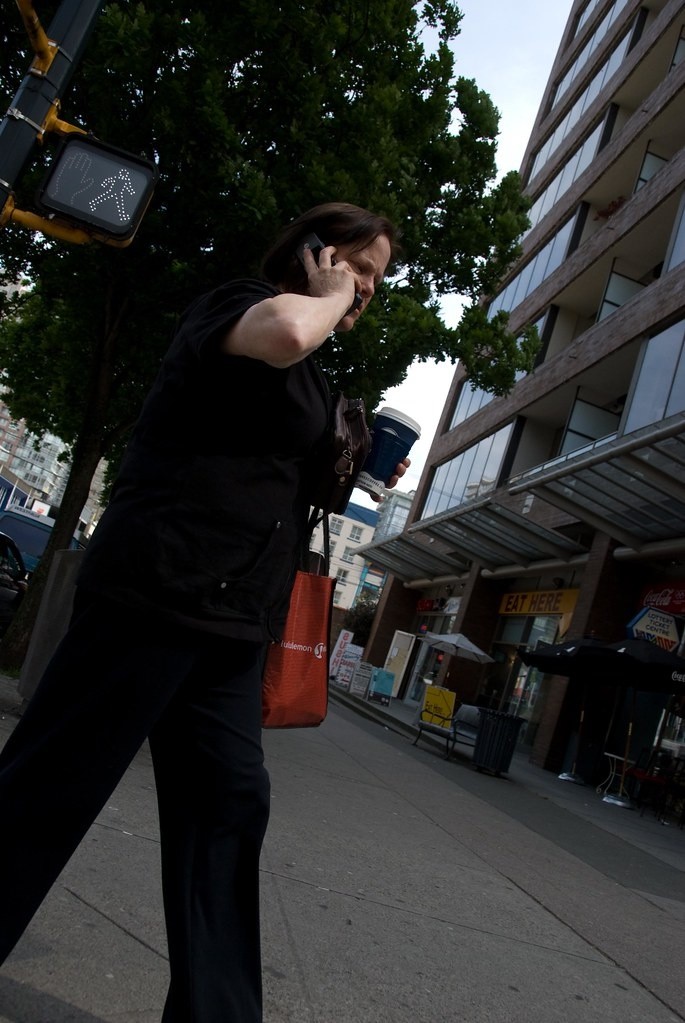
[1,502,92,581]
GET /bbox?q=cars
[0,529,30,630]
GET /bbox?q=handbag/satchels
[258,506,337,728]
[324,391,371,514]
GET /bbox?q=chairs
[628,742,685,830]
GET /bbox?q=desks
[595,750,636,799]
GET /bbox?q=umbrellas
[416,632,496,688]
[514,635,685,798]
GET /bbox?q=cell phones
[296,232,363,317]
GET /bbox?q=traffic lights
[39,129,162,252]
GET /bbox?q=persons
[1,201,411,1023]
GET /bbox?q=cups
[354,407,421,496]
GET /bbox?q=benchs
[412,704,482,763]
